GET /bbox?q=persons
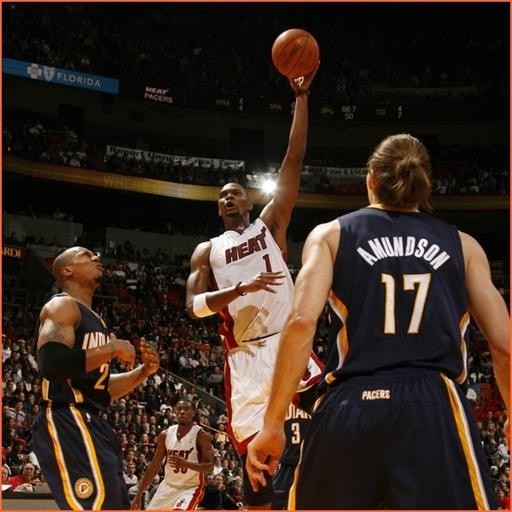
[469,291,512,510]
[186,59,322,510]
[4,199,225,237]
[29,247,159,511]
[247,132,512,511]
[2,230,65,494]
[92,239,338,511]
[4,2,509,193]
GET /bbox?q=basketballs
[272,29,321,80]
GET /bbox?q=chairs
[5,236,505,423]
[4,39,508,206]
